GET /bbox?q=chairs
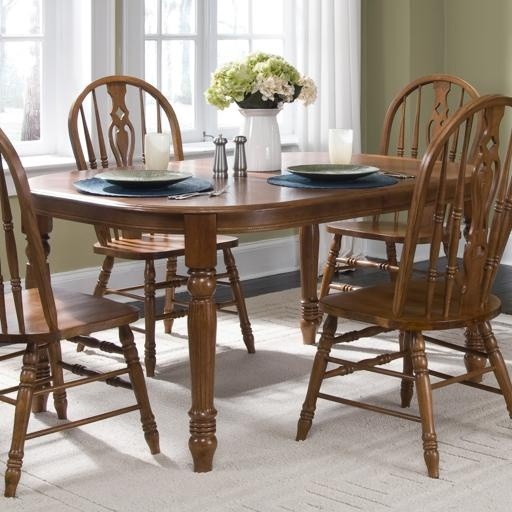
[296,93,511,477]
[320,74,480,351]
[69,76,255,375]
[1,127,160,498]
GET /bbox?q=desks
[19,153,485,471]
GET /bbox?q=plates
[94,169,192,188]
[287,164,380,183]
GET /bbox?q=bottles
[234,136,247,177]
[212,134,228,178]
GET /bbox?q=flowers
[204,52,317,111]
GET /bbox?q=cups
[144,133,171,170]
[328,128,354,165]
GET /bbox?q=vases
[240,110,282,171]
